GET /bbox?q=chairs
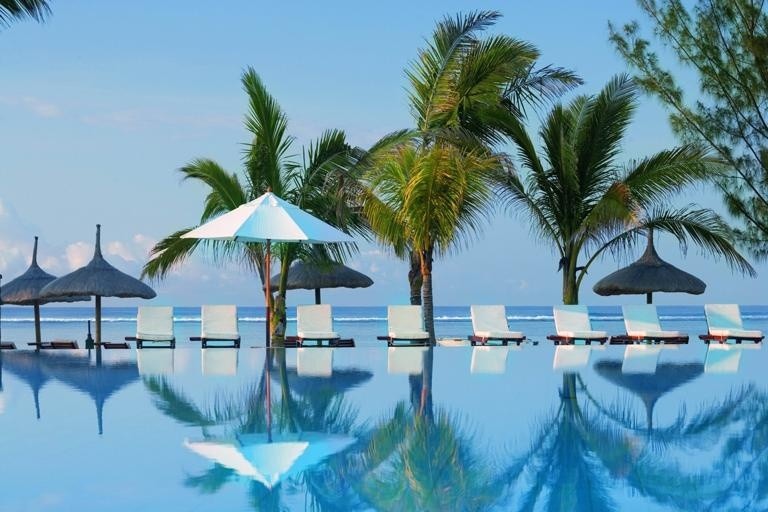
[125,304,176,349]
[50,341,78,348]
[286,304,341,348]
[377,305,430,347]
[103,342,130,349]
[190,304,240,349]
[0,340,16,347]
[609,304,689,345]
[699,303,765,344]
[468,304,526,345]
[546,304,608,345]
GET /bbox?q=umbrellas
[270,363,373,409]
[595,359,705,432]
[1,237,91,343]
[181,187,353,346]
[263,257,374,344]
[2,348,70,419]
[594,229,706,303]
[39,225,157,345]
[183,349,358,492]
[43,346,140,435]
